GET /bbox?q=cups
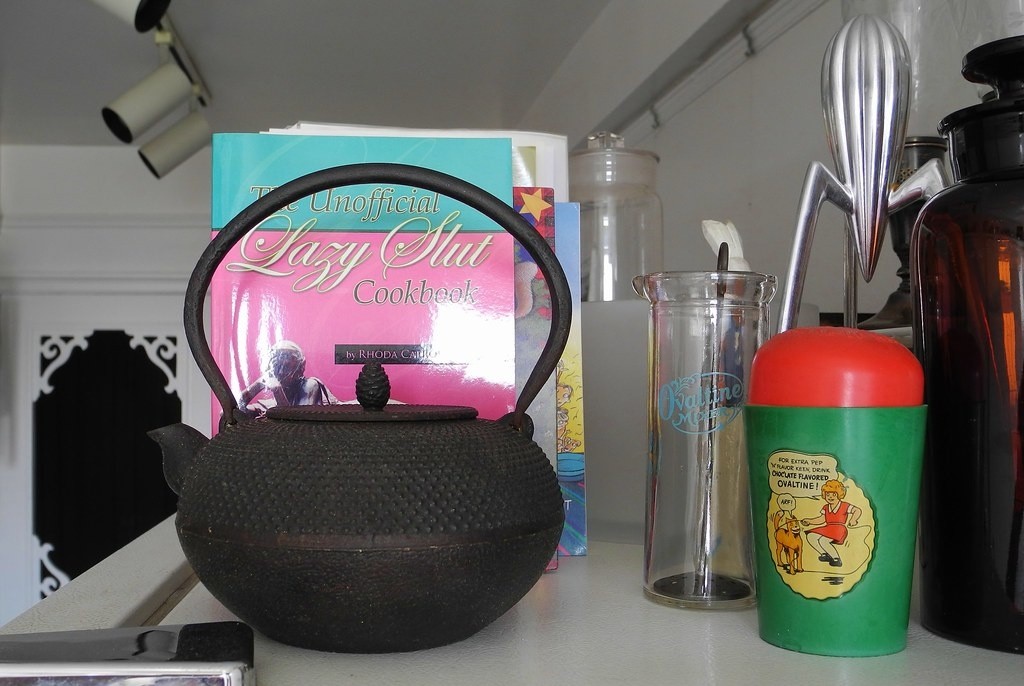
[741,399,929,662]
[569,123,664,543]
[632,265,783,609]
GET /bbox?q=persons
[219,339,405,419]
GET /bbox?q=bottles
[898,29,1023,656]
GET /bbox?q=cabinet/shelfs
[1,502,1024,686]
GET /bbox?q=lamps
[102,63,193,142]
[88,0,174,33]
[138,111,213,178]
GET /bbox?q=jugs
[147,157,572,656]
